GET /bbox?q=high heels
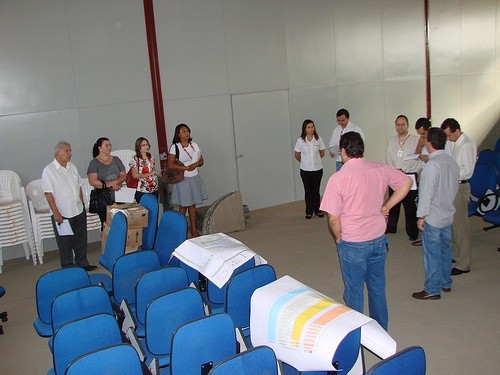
[190,230,200,237]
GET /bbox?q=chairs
[457,138,500,251]
[0,149,427,375]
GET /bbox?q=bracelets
[419,218,424,219]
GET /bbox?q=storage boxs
[101,203,148,254]
[196,205,211,230]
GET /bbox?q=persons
[330,108,365,172]
[294,119,326,219]
[320,131,414,332]
[86,137,127,232]
[128,137,159,249]
[42,140,97,271]
[412,127,460,300]
[168,123,209,237]
[385,114,476,275]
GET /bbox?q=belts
[458,180,470,184]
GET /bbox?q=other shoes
[452,259,455,263]
[412,240,422,246]
[412,292,440,300]
[442,288,451,292]
[306,215,311,219]
[83,265,98,271]
[316,213,324,217]
[450,268,470,275]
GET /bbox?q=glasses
[142,143,149,146]
[396,123,406,126]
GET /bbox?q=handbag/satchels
[125,158,140,188]
[89,181,113,213]
[160,143,185,184]
